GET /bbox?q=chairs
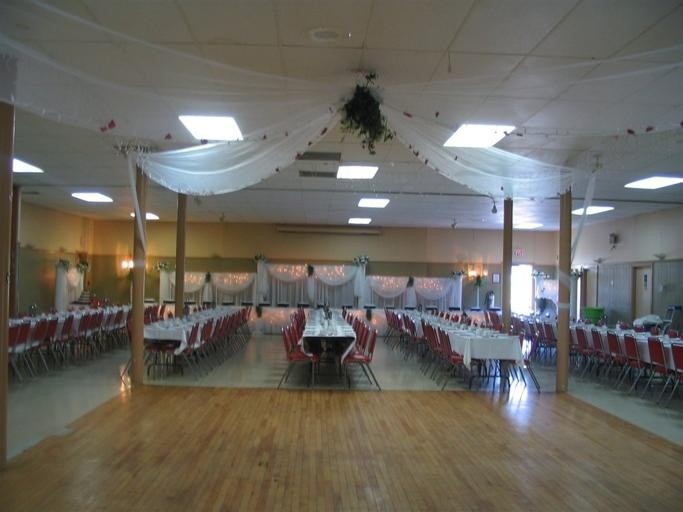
[617,334,642,394]
[641,336,670,404]
[382,307,426,360]
[7,302,167,384]
[341,306,382,391]
[126,303,170,383]
[277,305,312,388]
[602,331,627,391]
[415,318,470,392]
[589,328,605,379]
[177,304,252,365]
[526,321,557,361]
[489,312,504,332]
[575,325,592,376]
[667,343,683,404]
[511,316,525,356]
